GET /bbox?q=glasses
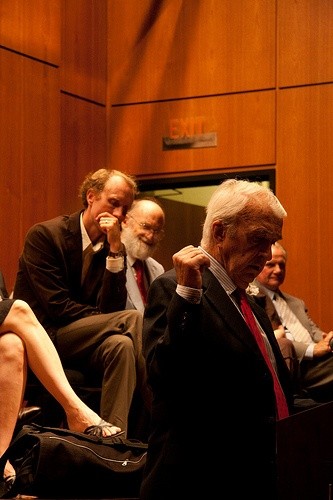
[129,213,165,241]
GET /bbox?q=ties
[234,289,288,420]
[274,292,315,344]
[132,257,150,309]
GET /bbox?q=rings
[106,220,107,223]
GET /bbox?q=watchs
[109,250,123,258]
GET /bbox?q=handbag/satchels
[0,424,148,500]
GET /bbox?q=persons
[0,167,166,500]
[142,177,333,500]
[249,241,333,399]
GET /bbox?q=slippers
[83,419,126,440]
